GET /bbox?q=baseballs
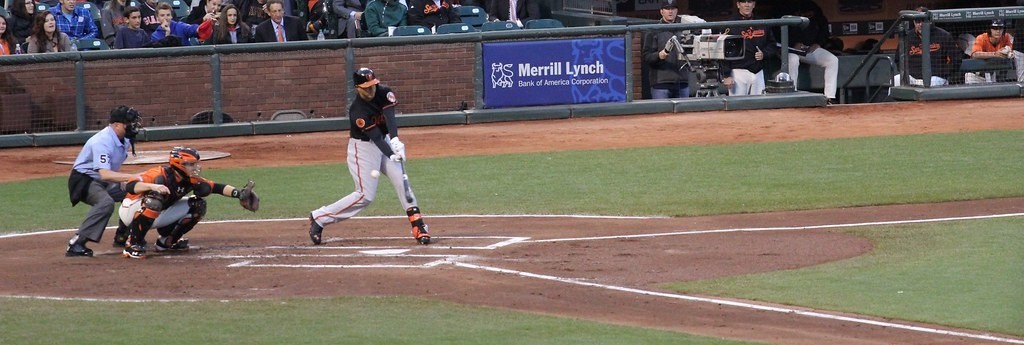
[371,170,379,178]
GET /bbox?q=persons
[971,17,1014,82]
[0,0,462,56]
[65,104,144,257]
[150,2,218,47]
[114,6,153,49]
[888,6,962,96]
[719,0,777,97]
[203,4,255,45]
[309,66,430,245]
[489,0,547,29]
[118,145,259,259]
[768,0,839,106]
[641,0,691,100]
[27,10,73,54]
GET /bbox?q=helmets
[110,105,140,124]
[987,18,1007,37]
[353,67,380,89]
[169,146,202,180]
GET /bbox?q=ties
[277,25,285,42]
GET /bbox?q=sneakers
[154,237,190,251]
[113,228,147,247]
[122,241,147,258]
[65,242,93,257]
[309,213,324,245]
[417,236,430,244]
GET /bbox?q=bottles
[15,44,21,54]
[317,30,326,41]
[70,43,77,52]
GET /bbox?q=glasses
[25,1,34,5]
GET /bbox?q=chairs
[392,6,564,35]
[190,111,234,125]
[272,109,307,121]
[0,0,190,50]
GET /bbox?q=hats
[913,7,930,23]
[198,18,213,40]
[661,0,680,9]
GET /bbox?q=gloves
[391,136,407,162]
[390,155,401,162]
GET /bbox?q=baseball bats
[400,159,413,203]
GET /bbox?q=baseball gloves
[241,181,260,211]
